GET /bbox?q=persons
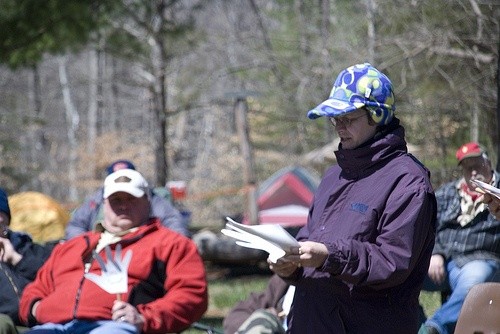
[418,142,500,334]
[18,168,208,334]
[65,159,190,238]
[267,63,437,333]
[222,274,295,334]
[0,189,51,334]
[454,192,500,334]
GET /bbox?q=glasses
[329,114,367,127]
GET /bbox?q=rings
[298,247,302,254]
[120,316,125,321]
[298,262,301,268]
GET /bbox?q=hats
[103,168,149,200]
[0,186,11,223]
[456,143,481,167]
[307,62,396,125]
